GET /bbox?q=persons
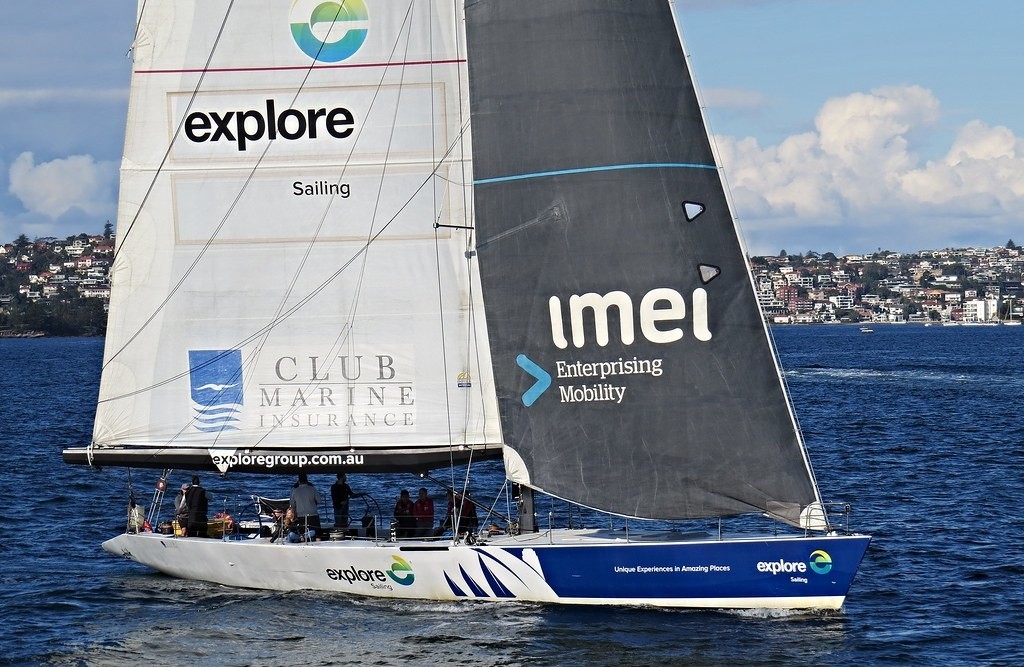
[174,476,213,537]
[434,488,477,541]
[330,473,364,527]
[269,508,315,542]
[290,474,322,542]
[413,488,434,538]
[392,489,414,538]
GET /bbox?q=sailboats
[63,0,874,615]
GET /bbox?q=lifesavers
[213,512,233,528]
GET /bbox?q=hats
[181,483,190,489]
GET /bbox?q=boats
[942,321,959,327]
[925,323,932,326]
[961,321,997,327]
[859,328,868,330]
[861,329,873,333]
